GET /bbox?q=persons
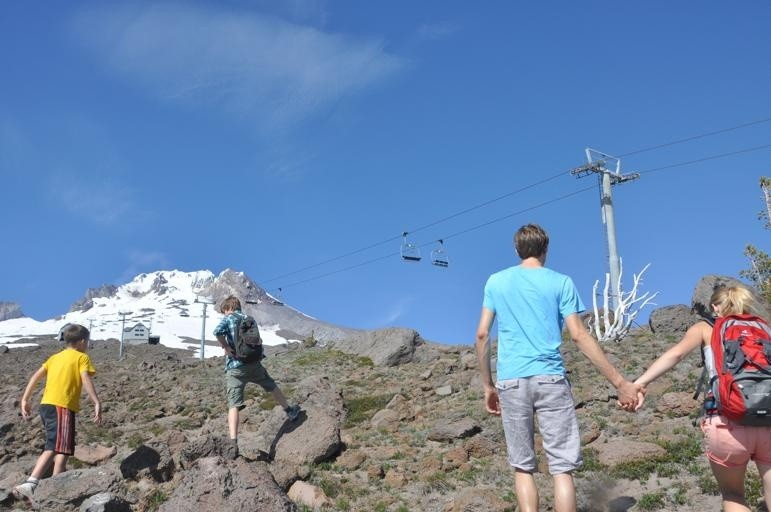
[11,324,104,491]
[615,286,771,512]
[475,223,647,511]
[212,295,304,457]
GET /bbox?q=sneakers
[289,405,301,422]
[12,486,38,509]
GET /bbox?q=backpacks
[228,313,262,363]
[703,311,771,425]
